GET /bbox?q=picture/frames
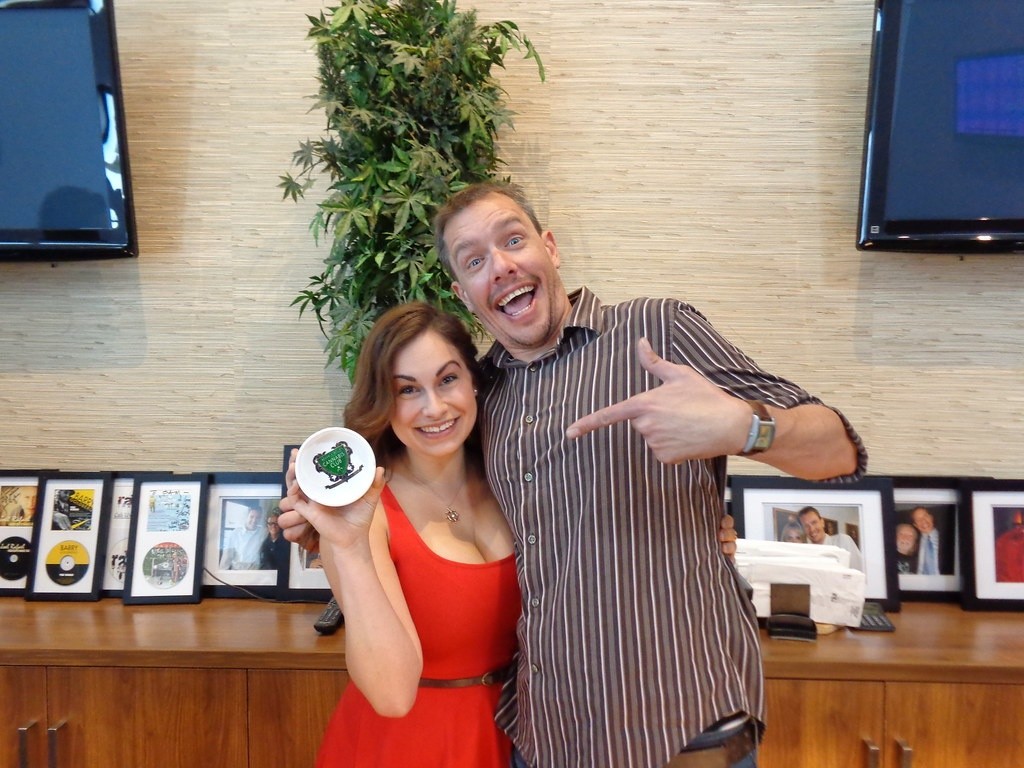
[0,444,333,607]
[723,475,1024,612]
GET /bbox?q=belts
[668,719,766,768]
[420,666,517,687]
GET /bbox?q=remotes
[314,596,342,634]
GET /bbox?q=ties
[922,535,936,575]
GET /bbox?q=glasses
[62,490,72,496]
[268,522,278,526]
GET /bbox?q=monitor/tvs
[0,0,139,261]
[857,0,1024,256]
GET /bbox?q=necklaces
[396,455,466,522]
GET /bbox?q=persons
[278,184,871,767]
[259,512,280,569]
[797,506,861,571]
[897,505,955,575]
[286,304,737,767]
[52,489,77,530]
[221,507,267,569]
[779,521,808,543]
[9,487,38,524]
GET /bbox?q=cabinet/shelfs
[0,594,1024,768]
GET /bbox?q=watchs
[735,397,775,457]
